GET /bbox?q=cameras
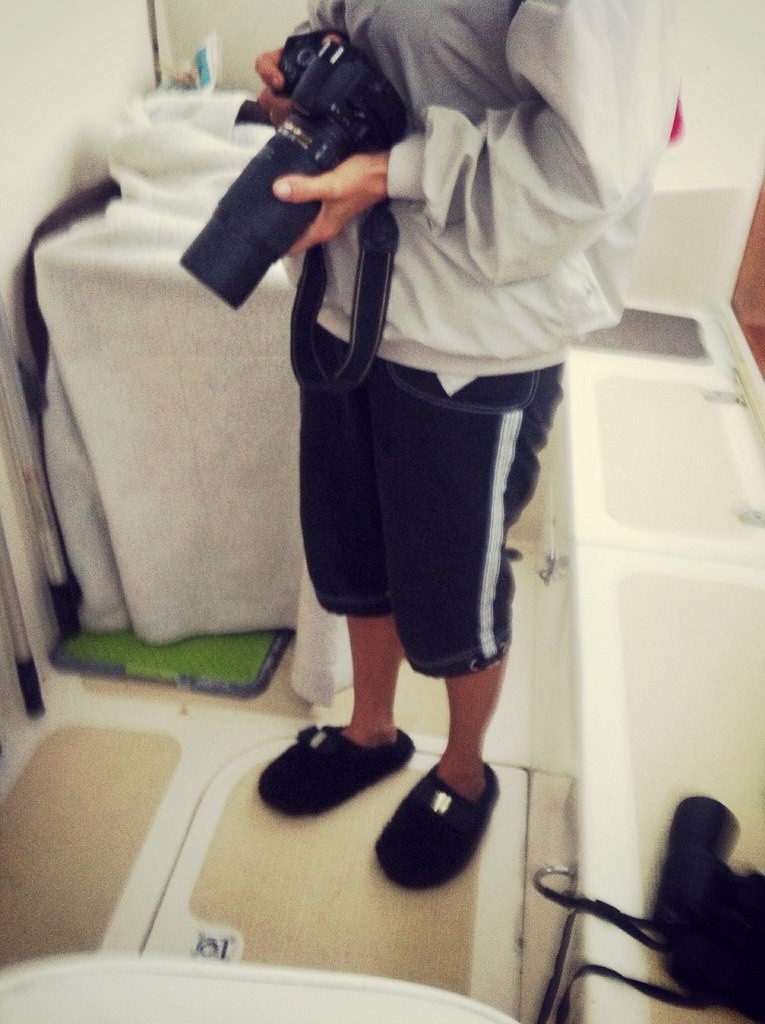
[178,39,405,313]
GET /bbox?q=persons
[256,0,656,892]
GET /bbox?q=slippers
[374,756,498,888]
[257,724,415,815]
